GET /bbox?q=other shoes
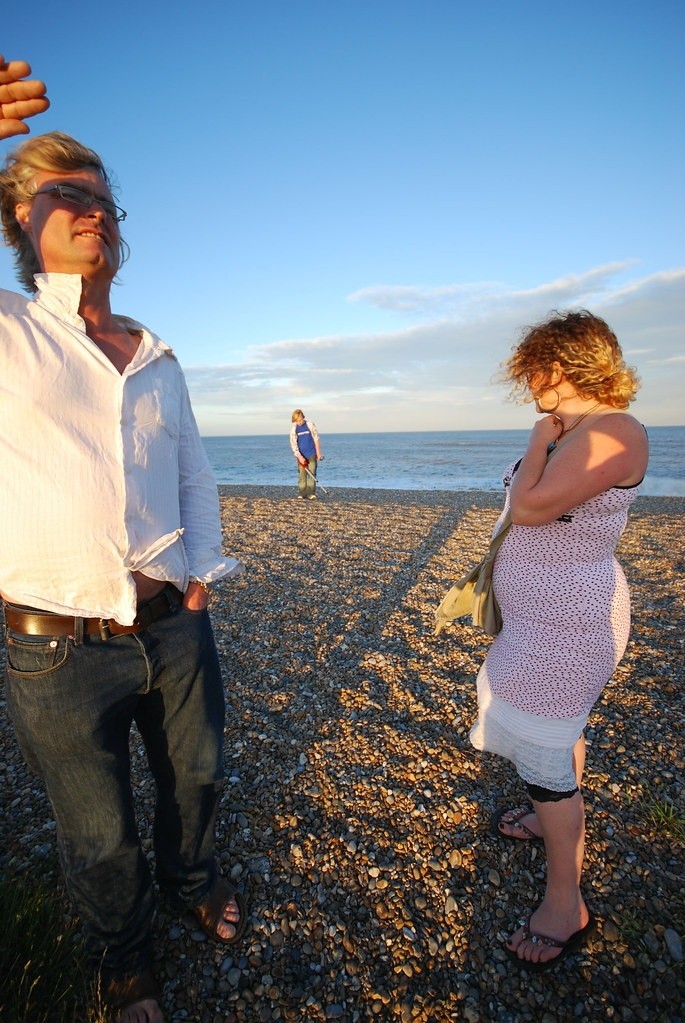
[307,494,316,500]
[298,496,303,500]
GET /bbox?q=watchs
[189,580,214,594]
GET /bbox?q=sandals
[96,967,167,1023]
[193,877,248,944]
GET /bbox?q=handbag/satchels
[431,553,501,638]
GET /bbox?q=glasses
[29,183,127,222]
[319,456,324,461]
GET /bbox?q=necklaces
[547,401,603,453]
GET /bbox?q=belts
[4,587,183,642]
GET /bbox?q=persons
[468,309,649,971]
[290,409,323,499]
[0,54,248,1022]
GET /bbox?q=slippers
[492,804,544,841]
[502,910,597,972]
